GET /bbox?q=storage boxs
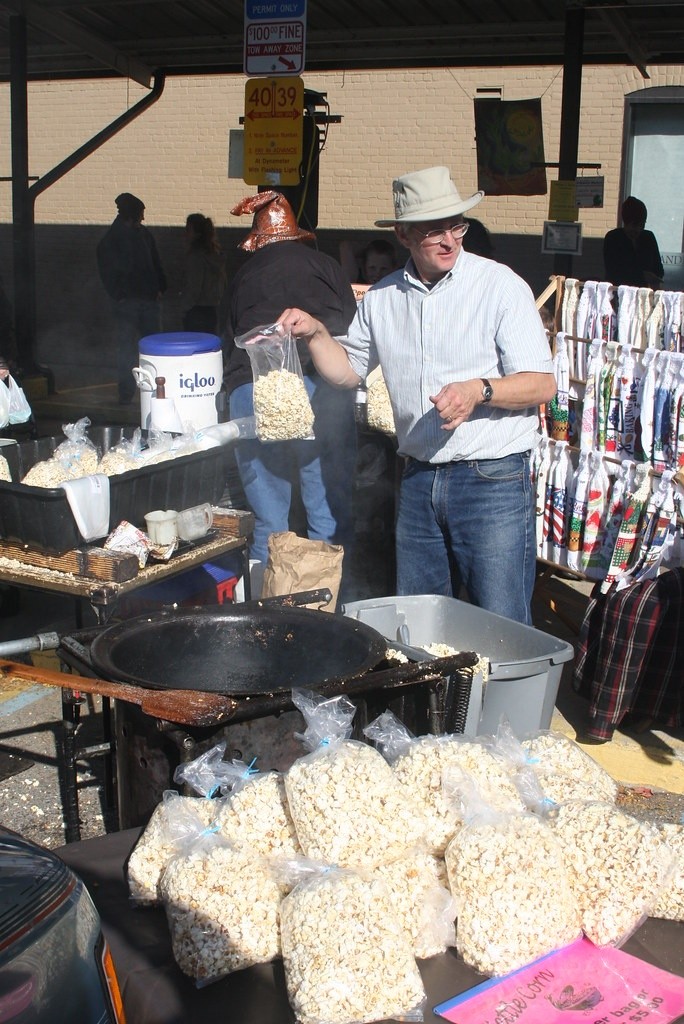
[0,425,239,549]
[339,594,574,744]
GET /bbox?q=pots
[90,603,391,697]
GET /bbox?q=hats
[374,166,485,228]
[115,193,145,220]
[622,197,646,225]
[230,190,316,253]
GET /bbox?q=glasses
[409,217,470,244]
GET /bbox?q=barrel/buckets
[131,330,224,434]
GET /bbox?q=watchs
[480,378,494,406]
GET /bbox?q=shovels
[0,658,238,726]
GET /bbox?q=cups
[177,504,215,542]
[145,507,182,549]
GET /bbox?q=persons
[96,192,168,407]
[361,239,396,286]
[275,166,557,627]
[603,195,665,342]
[167,214,229,332]
[224,190,359,603]
